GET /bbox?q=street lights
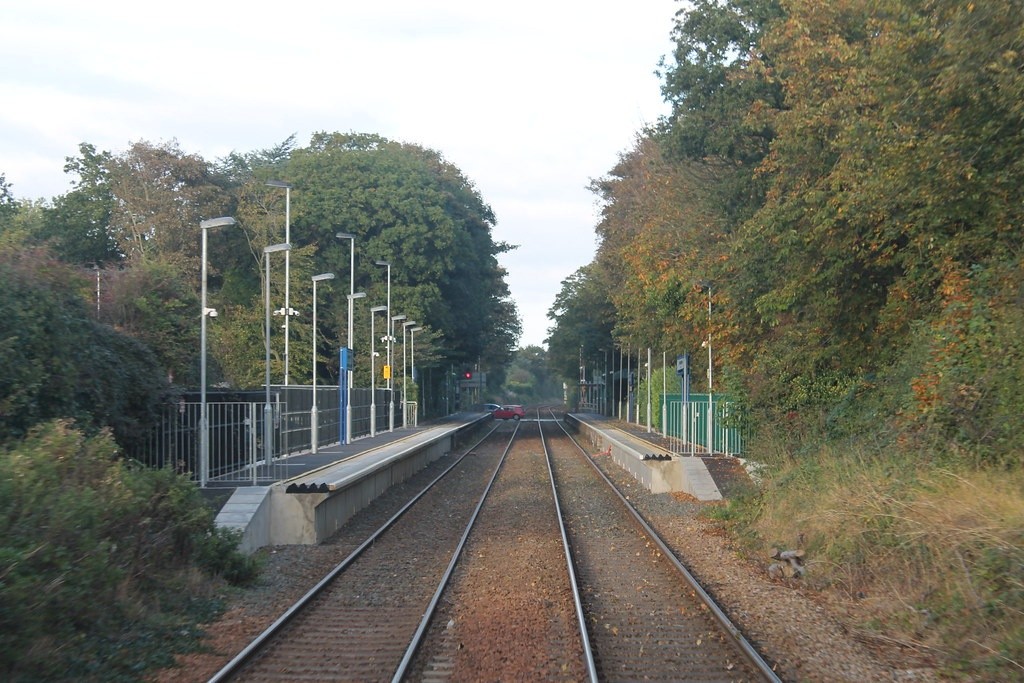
[197,216,236,484]
[613,342,622,420]
[411,327,422,380]
[389,314,406,432]
[346,293,367,444]
[262,244,292,466]
[696,280,712,454]
[265,180,292,386]
[599,348,607,416]
[370,306,387,438]
[335,231,355,388]
[376,260,391,389]
[402,321,416,428]
[311,273,334,454]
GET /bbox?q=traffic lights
[465,372,471,380]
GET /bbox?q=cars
[490,405,526,420]
[484,404,504,412]
